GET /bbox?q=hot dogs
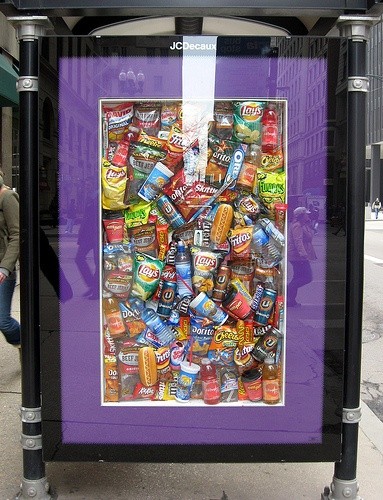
[209,204,233,244]
[139,347,157,387]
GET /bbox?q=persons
[0,172,22,363]
[314,207,319,230]
[65,199,76,234]
[40,228,73,304]
[287,207,314,307]
[74,176,100,302]
[372,198,381,219]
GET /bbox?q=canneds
[254,287,277,326]
[157,195,186,230]
[212,264,231,301]
[156,280,177,317]
[250,326,283,362]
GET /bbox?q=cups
[175,362,201,402]
[137,162,175,203]
[103,210,125,245]
[223,288,252,319]
[242,369,263,402]
[189,292,230,325]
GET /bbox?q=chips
[234,124,260,144]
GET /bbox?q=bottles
[138,305,176,346]
[201,358,220,404]
[111,126,142,167]
[261,101,280,153]
[104,293,127,339]
[175,241,194,297]
[243,214,285,268]
[238,142,263,194]
[262,357,280,404]
